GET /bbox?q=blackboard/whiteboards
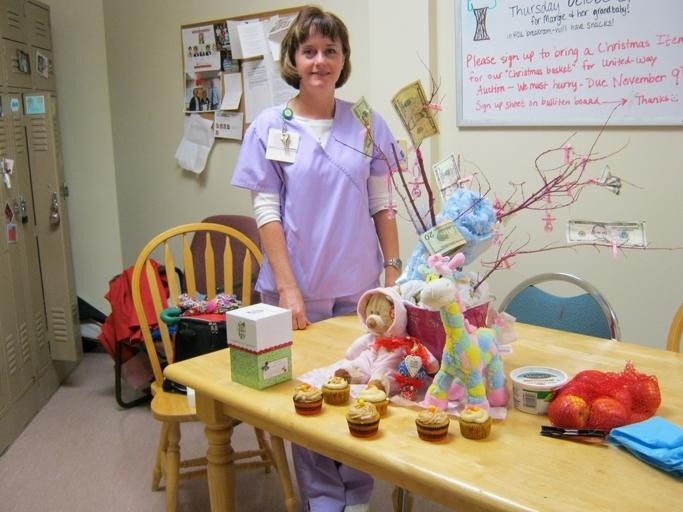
[453,0,683,129]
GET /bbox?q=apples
[549,369,664,430]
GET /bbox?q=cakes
[346,400,381,437]
[415,406,450,443]
[459,404,490,441]
[293,383,323,416]
[322,376,350,406]
[356,384,387,419]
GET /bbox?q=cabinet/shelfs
[0,0,84,456]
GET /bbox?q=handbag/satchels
[162,317,230,395]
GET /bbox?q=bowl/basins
[509,366,568,417]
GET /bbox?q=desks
[163,310,683,512]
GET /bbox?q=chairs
[114,214,263,410]
[665,304,683,353]
[498,272,623,342]
[131,223,301,512]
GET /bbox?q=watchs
[382,259,403,270]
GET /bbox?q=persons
[39,57,44,73]
[408,109,429,130]
[190,89,209,110]
[215,26,229,45]
[591,223,609,242]
[187,33,212,57]
[230,7,406,511]
[442,182,460,197]
[223,52,231,72]
[21,54,29,72]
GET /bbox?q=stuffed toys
[421,252,510,410]
[332,287,440,393]
